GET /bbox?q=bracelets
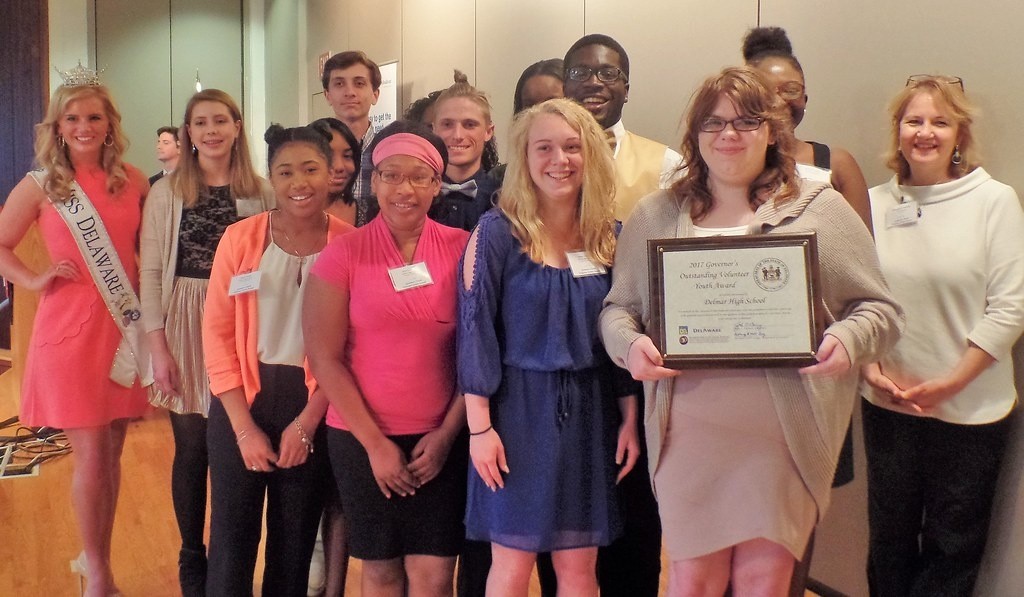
[293,418,314,447]
[469,425,492,435]
[235,425,261,443]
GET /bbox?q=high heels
[77,551,124,597]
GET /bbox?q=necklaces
[904,174,950,217]
[278,211,329,287]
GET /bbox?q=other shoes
[303,528,327,597]
[178,545,209,597]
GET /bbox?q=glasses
[374,166,436,189]
[904,73,964,96]
[775,81,806,100]
[563,65,629,83]
[698,116,766,133]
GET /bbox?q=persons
[203,29,904,597]
[0,60,150,597]
[857,77,1024,597]
[139,87,279,597]
[147,126,185,187]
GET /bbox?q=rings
[57,265,60,270]
[252,465,258,471]
